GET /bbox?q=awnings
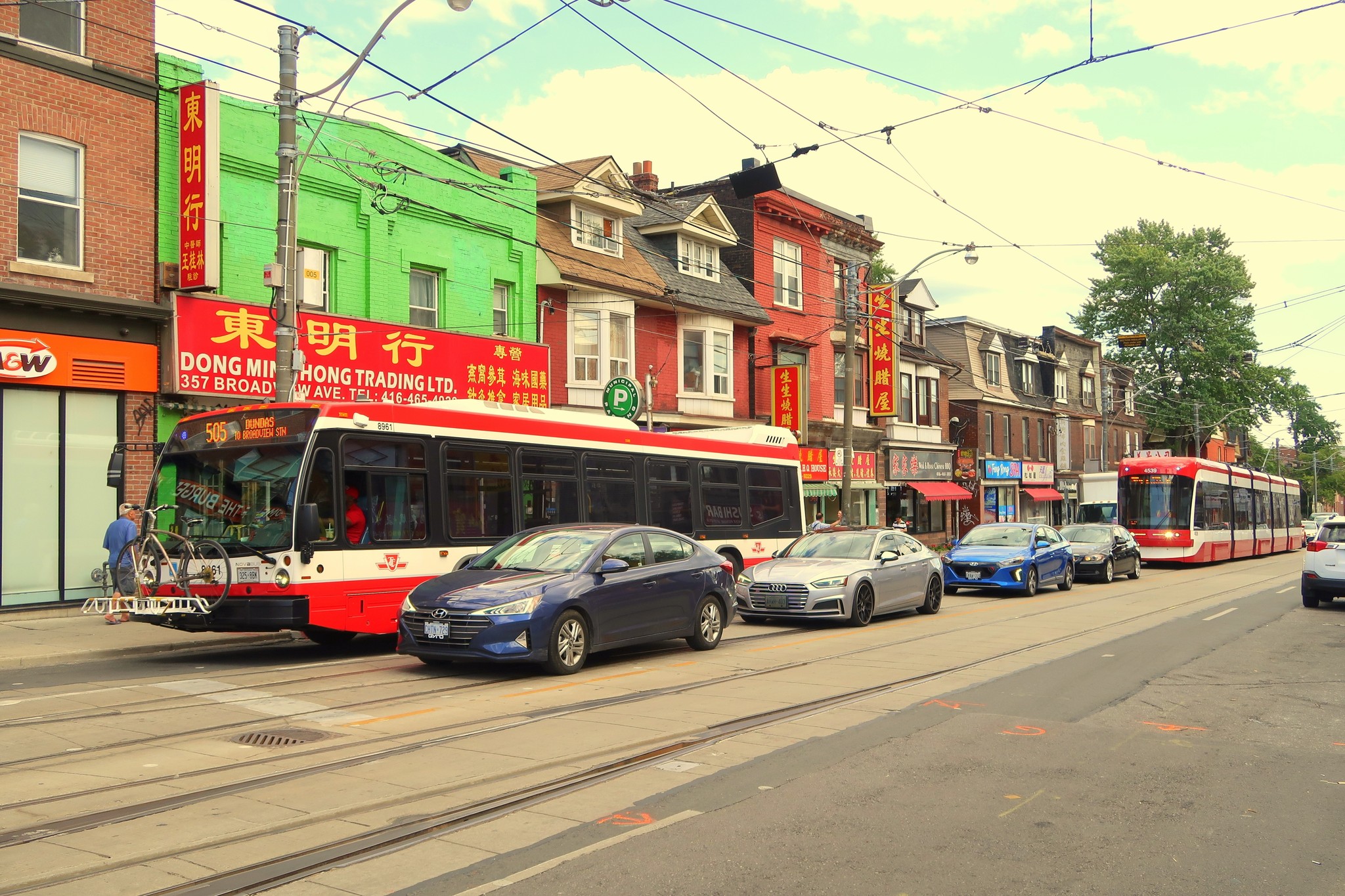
[906,482,973,502]
[835,483,889,489]
[1023,488,1064,501]
[803,483,838,497]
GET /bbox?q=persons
[837,510,843,525]
[103,502,142,624]
[318,484,370,545]
[808,513,843,531]
[892,513,907,533]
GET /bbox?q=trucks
[1067,472,1119,525]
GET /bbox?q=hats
[344,484,359,499]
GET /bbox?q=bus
[1116,456,1302,566]
[80,399,809,650]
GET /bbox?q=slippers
[118,619,129,622]
[106,618,121,624]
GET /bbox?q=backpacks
[806,522,821,533]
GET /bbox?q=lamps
[949,417,959,424]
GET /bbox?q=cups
[325,528,334,542]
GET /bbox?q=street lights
[1193,403,1257,458]
[1313,450,1345,513]
[274,0,479,400]
[1276,436,1320,478]
[1100,365,1183,473]
[1243,427,1292,470]
[840,240,980,526]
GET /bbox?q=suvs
[1301,513,1345,608]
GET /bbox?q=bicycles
[115,503,232,612]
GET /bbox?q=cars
[1301,523,1307,548]
[1058,523,1142,584]
[735,524,945,627]
[395,523,738,676]
[1308,512,1339,526]
[1301,519,1320,538]
[940,522,1075,598]
